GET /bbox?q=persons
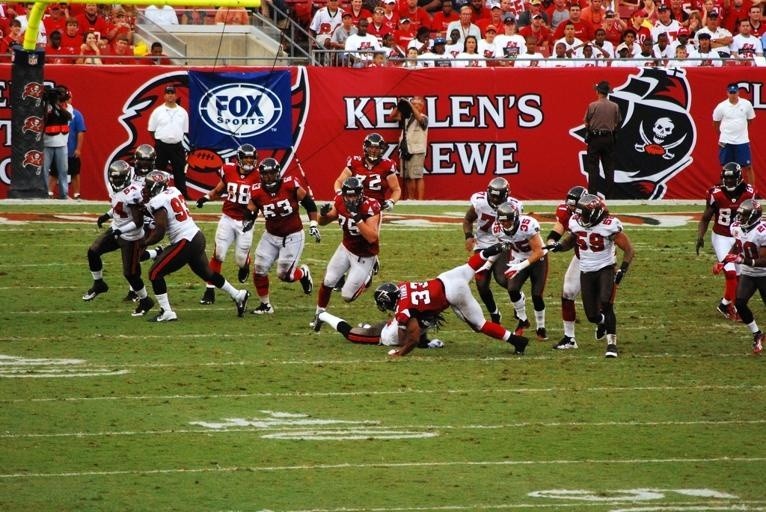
[42,85,73,202]
[82,144,322,322]
[264,1,765,66]
[712,198,765,354]
[713,83,757,191]
[585,81,622,198]
[48,89,84,201]
[0,1,249,66]
[147,85,191,202]
[308,177,636,360]
[389,96,429,199]
[313,133,402,332]
[695,161,758,322]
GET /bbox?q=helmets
[258,158,281,189]
[486,176,509,210]
[237,143,258,175]
[342,177,364,208]
[140,169,170,198]
[566,185,608,228]
[497,202,520,236]
[735,198,762,229]
[720,162,742,192]
[108,160,131,193]
[362,133,386,164]
[134,144,158,176]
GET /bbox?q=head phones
[56,85,69,97]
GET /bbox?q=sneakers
[299,263,313,294]
[230,289,251,317]
[751,330,765,354]
[82,278,109,301]
[486,240,512,256]
[250,302,274,315]
[535,327,549,341]
[200,288,215,305]
[553,335,579,350]
[309,308,326,332]
[595,314,619,358]
[332,254,381,292]
[145,307,178,322]
[238,255,251,283]
[489,293,532,357]
[121,289,155,317]
[716,297,741,323]
[755,192,763,200]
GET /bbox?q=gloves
[344,200,363,225]
[614,261,629,286]
[242,210,256,232]
[711,260,728,275]
[724,254,745,265]
[541,242,563,253]
[503,259,530,280]
[308,225,321,243]
[320,202,332,216]
[97,212,123,246]
[194,195,211,208]
[475,260,492,274]
[382,198,395,211]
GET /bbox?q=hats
[384,0,396,4]
[592,80,614,94]
[400,17,412,24]
[531,0,541,6]
[502,12,515,23]
[342,12,352,17]
[530,5,720,54]
[491,2,501,9]
[727,84,739,92]
[165,86,176,94]
